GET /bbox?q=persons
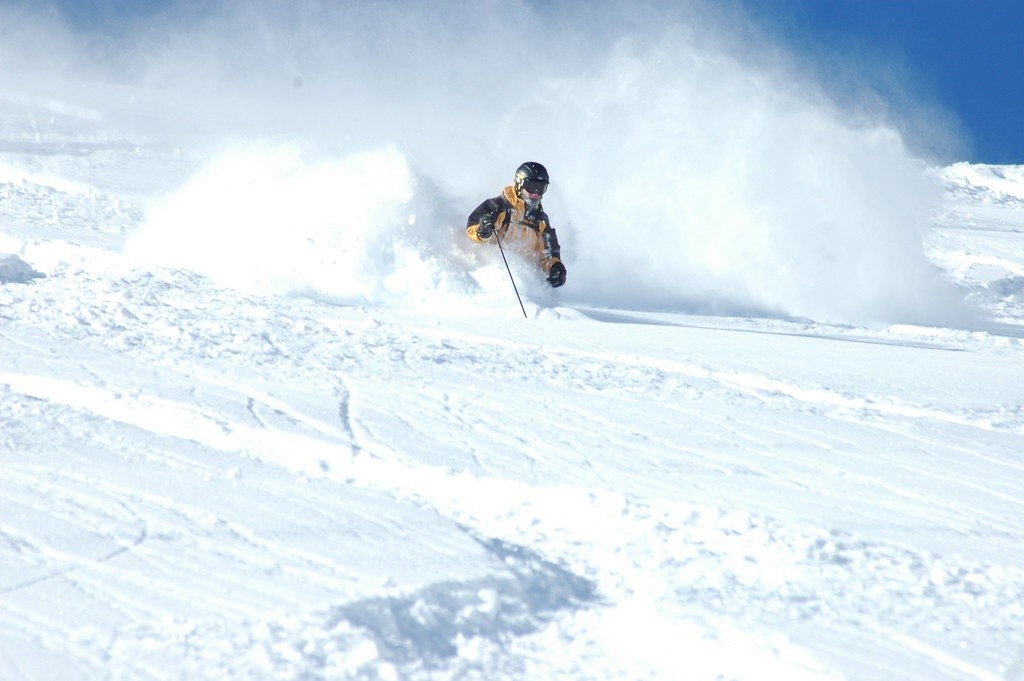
[466,162,567,288]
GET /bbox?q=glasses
[524,179,548,195]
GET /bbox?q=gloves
[478,213,496,238]
[546,262,566,288]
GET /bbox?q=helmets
[514,162,549,196]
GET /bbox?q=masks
[521,194,541,209]
